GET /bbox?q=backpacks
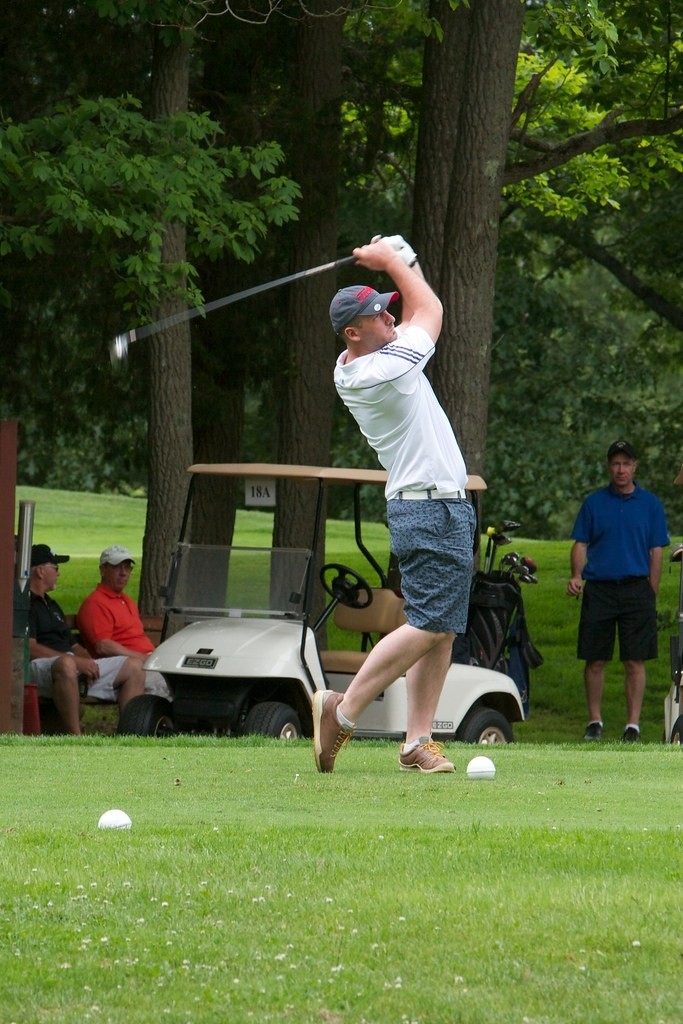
[451,570,544,719]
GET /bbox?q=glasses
[45,564,58,572]
[103,564,133,572]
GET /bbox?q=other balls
[97,809,132,830]
[466,755,497,781]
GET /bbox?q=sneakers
[623,727,640,743]
[312,689,354,774]
[399,733,456,773]
[583,722,602,741]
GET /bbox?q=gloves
[371,234,417,265]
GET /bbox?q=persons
[78,545,172,701]
[566,440,669,744]
[22,544,147,737]
[311,234,478,773]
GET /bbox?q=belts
[394,488,466,500]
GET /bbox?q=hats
[329,284,399,335]
[30,544,70,568]
[607,440,637,460]
[100,545,136,567]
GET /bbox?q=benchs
[40,614,170,703]
[319,587,405,676]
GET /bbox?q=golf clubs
[498,551,520,577]
[106,252,359,379]
[499,556,539,588]
[483,519,522,576]
